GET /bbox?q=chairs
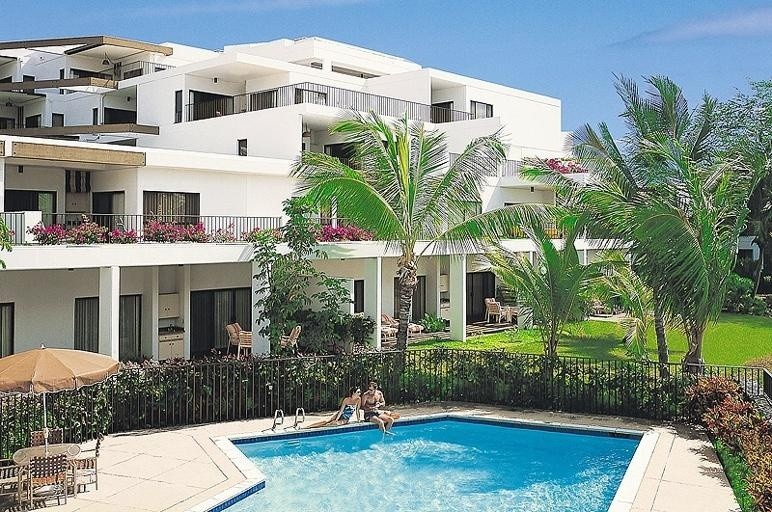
[380,312,424,343]
[0,428,104,512]
[590,294,627,314]
[226,322,302,360]
[484,298,520,324]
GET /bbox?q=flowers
[22,210,375,246]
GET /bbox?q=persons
[301,386,362,428]
[362,381,396,437]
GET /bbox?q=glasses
[357,391,361,393]
[368,389,372,391]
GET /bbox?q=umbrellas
[1,342,120,458]
